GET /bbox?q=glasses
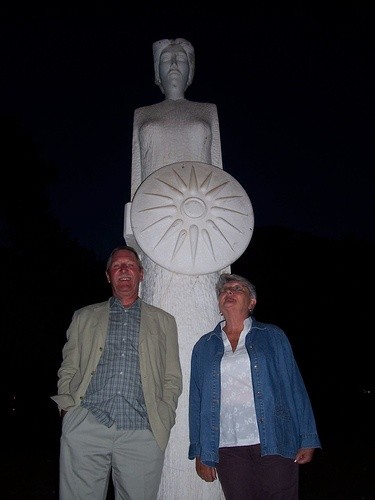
[219,285,252,296]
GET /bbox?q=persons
[49,244,186,500]
[118,34,258,500]
[185,270,322,500]
